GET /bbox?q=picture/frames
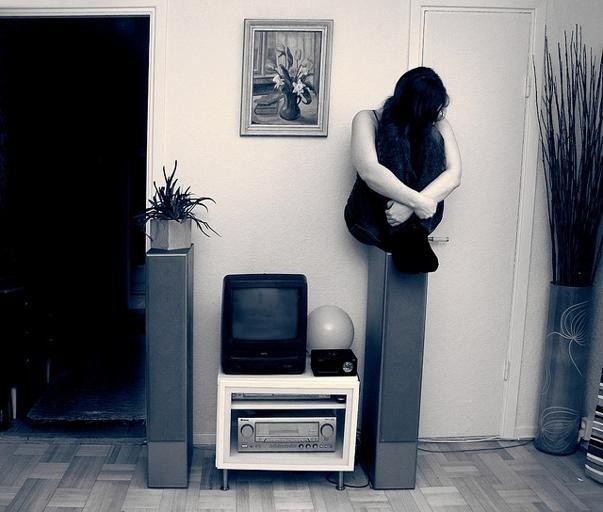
[239,18,334,137]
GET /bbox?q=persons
[342,68,464,276]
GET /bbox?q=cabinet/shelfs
[215,372,361,492]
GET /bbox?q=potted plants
[135,160,222,252]
[532,24,603,457]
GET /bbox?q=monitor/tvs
[220,274,307,375]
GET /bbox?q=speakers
[360,243,429,491]
[144,243,196,489]
